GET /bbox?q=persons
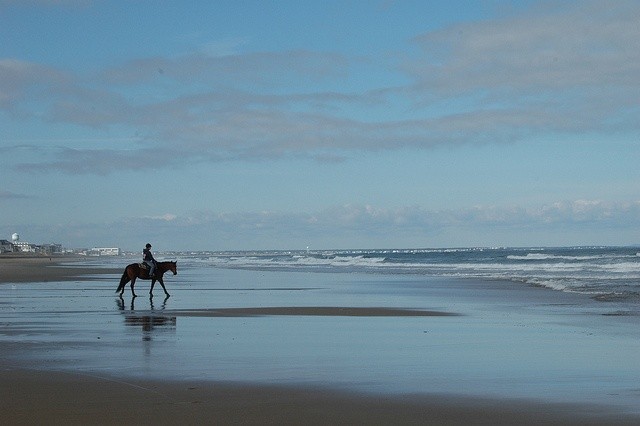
[141,242,156,278]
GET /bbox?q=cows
[115,261,177,296]
